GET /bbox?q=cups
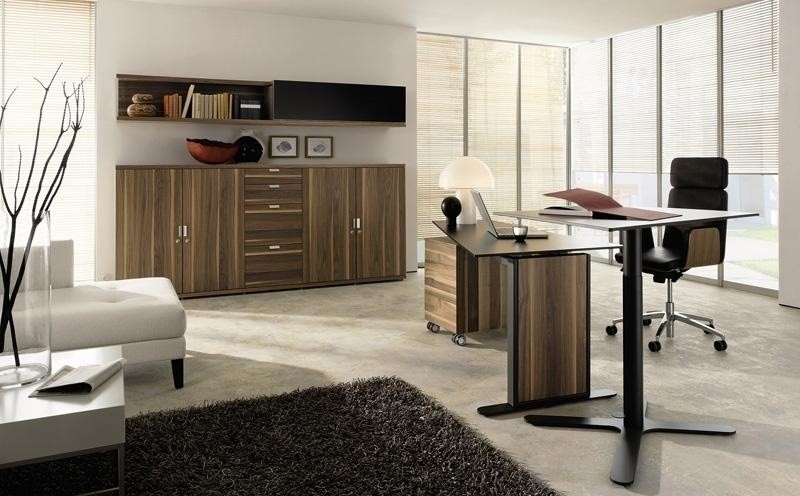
[513,225,529,245]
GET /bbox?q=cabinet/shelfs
[115,75,272,124]
[309,164,405,287]
[244,163,308,294]
[273,78,407,127]
[116,165,244,297]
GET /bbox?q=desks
[433,219,625,416]
[493,207,759,486]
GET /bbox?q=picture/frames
[305,136,333,158]
[269,136,298,158]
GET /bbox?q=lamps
[438,157,494,225]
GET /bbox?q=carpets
[0,376,562,495]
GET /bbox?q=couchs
[0,277,188,390]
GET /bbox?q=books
[163,84,240,119]
[538,188,682,221]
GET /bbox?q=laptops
[471,189,549,241]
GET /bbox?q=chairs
[605,156,729,352]
